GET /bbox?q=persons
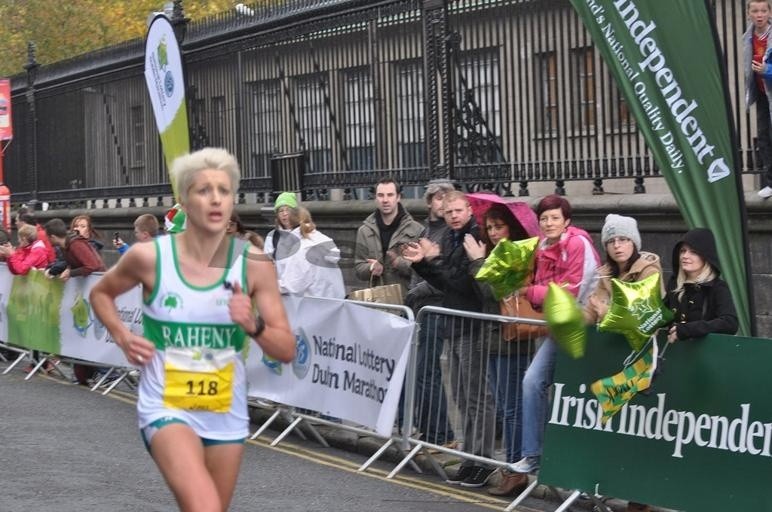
[227,191,345,429]
[354,179,735,512]
[1,208,110,386]
[742,0,772,197]
[112,214,159,256]
[88,147,296,512]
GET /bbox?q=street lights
[499,295,548,341]
[349,267,403,315]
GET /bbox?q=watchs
[246,315,266,337]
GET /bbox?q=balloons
[474,237,541,301]
[543,281,586,359]
[599,273,674,354]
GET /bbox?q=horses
[114,232,119,244]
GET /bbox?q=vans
[488,473,528,496]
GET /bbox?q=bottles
[600,214,641,252]
[425,179,455,205]
[274,192,297,212]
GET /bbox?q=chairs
[511,457,540,473]
[448,465,498,487]
[46,358,61,372]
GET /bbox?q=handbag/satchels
[246,315,266,337]
